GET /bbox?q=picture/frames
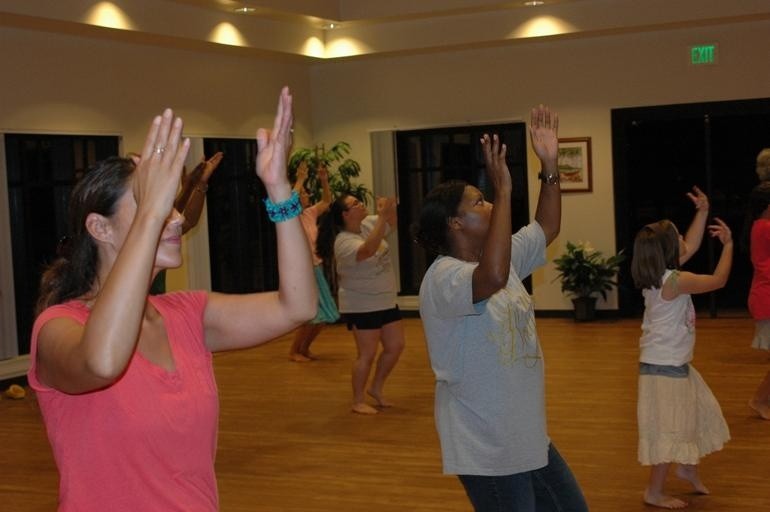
[555,136,592,194]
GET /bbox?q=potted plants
[547,238,633,324]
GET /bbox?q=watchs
[537,168,560,186]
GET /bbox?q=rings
[153,145,165,155]
[290,127,296,133]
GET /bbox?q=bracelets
[263,189,303,223]
[196,182,209,193]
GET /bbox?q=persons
[148,151,224,294]
[744,148,770,420]
[412,103,587,511]
[332,193,408,417]
[626,185,733,510]
[25,85,320,511]
[286,159,341,363]
